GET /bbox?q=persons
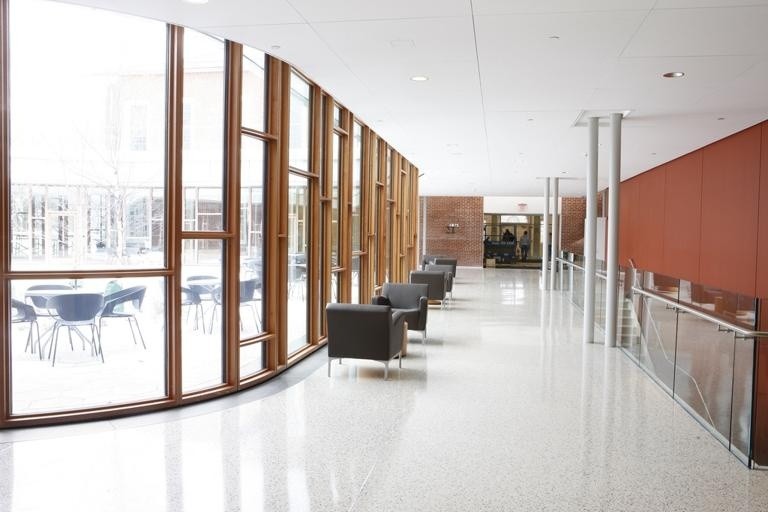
[503,229,515,241]
[548,232,552,261]
[519,230,530,262]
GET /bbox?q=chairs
[11,257,306,368]
[324,254,458,379]
